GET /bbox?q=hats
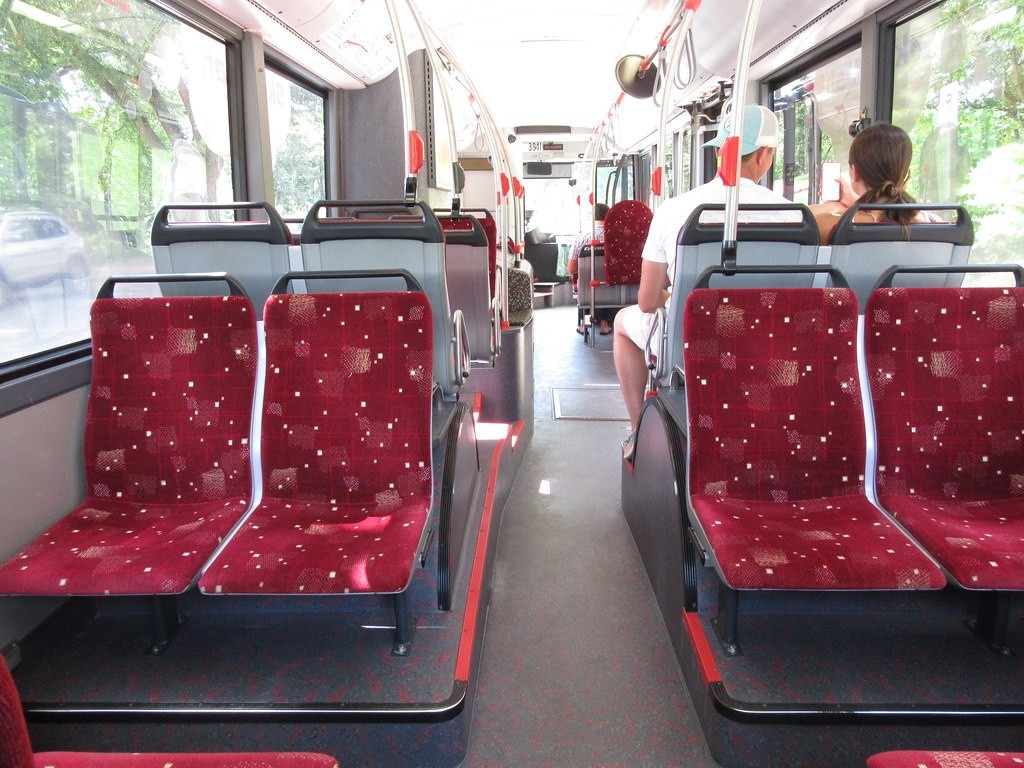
[702,103,781,159]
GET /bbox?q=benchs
[282,213,502,368]
[643,202,975,395]
[1,268,433,657]
[681,263,1024,656]
[151,199,471,400]
[351,206,500,334]
[576,243,640,345]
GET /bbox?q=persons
[807,123,945,246]
[567,203,612,336]
[613,103,803,458]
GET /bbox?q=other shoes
[621,427,639,459]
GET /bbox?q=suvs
[0,211,84,294]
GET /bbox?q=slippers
[576,326,590,336]
[598,327,612,336]
